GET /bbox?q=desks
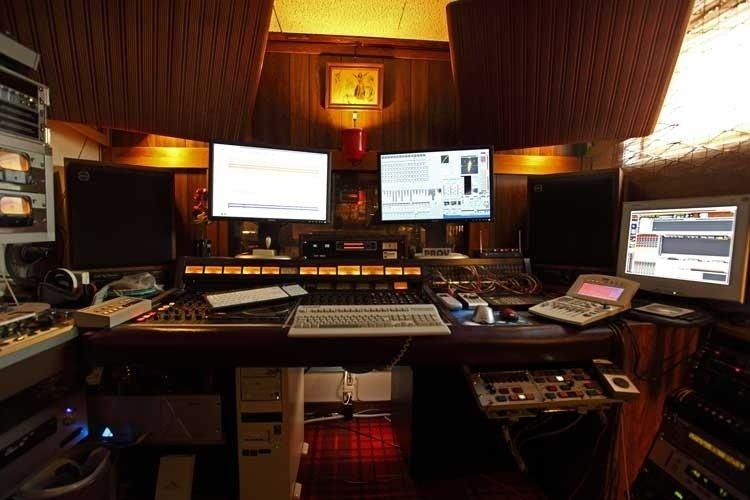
[76,318,632,500]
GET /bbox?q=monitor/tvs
[208,140,332,225]
[525,167,622,286]
[63,156,175,276]
[616,194,750,304]
[377,144,495,225]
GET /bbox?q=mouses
[500,306,518,322]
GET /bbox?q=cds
[647,305,683,315]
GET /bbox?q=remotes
[436,292,463,311]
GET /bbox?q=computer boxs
[236,366,309,500]
[87,378,228,445]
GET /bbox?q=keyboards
[287,304,451,338]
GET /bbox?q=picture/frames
[323,64,384,113]
